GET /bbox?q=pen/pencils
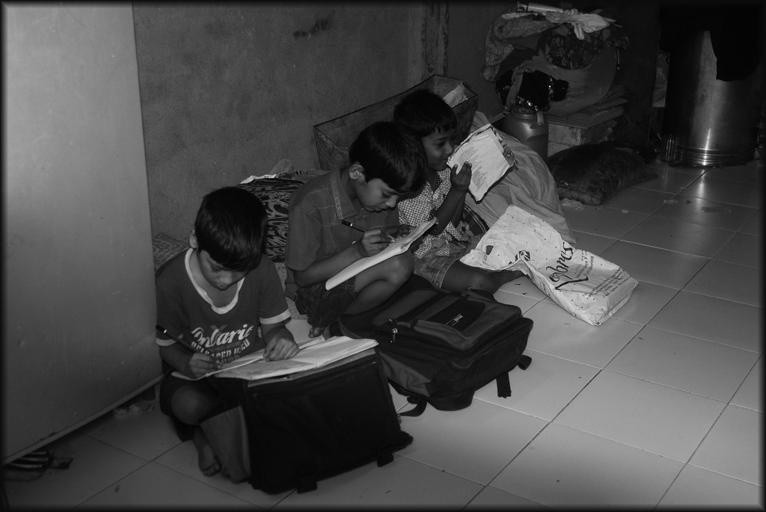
[153,325,221,369]
[341,219,394,243]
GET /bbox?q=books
[446,124,515,204]
[172,334,325,382]
[325,218,435,291]
[215,336,379,381]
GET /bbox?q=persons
[391,87,526,296]
[153,184,301,478]
[281,119,426,341]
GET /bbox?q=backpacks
[339,273,539,416]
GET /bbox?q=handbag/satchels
[459,203,640,328]
[240,347,419,498]
